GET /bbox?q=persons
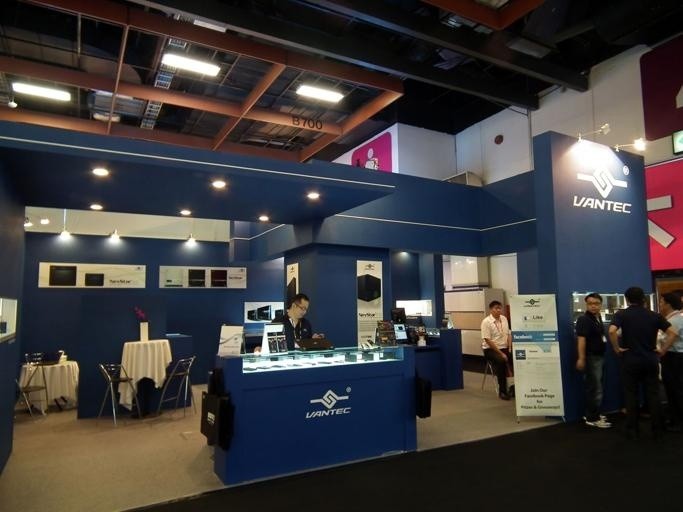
[480,300,515,401]
[608,287,683,445]
[254,293,325,353]
[575,292,613,429]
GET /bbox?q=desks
[117,339,172,418]
[20,361,81,411]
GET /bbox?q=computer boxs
[358,274,381,302]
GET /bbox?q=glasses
[296,304,309,312]
[587,301,601,306]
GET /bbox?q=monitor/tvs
[188,269,206,287]
[257,305,271,320]
[210,269,227,287]
[86,273,104,286]
[394,324,409,342]
[248,310,257,320]
[50,265,76,287]
[394,299,433,318]
[286,277,296,302]
[391,308,405,322]
[275,310,283,319]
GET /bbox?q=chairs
[157,356,196,417]
[95,363,141,425]
[14,363,49,419]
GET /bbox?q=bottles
[24,354,29,365]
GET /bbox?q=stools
[480,359,514,397]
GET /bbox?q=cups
[32,353,42,364]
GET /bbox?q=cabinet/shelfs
[207,345,416,486]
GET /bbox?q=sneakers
[583,414,607,420]
[585,420,612,428]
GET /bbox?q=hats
[661,292,681,306]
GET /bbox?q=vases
[141,322,148,341]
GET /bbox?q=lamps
[12,76,71,104]
[614,138,645,152]
[24,217,33,228]
[60,229,71,239]
[186,234,196,245]
[578,124,610,142]
[109,229,121,243]
[295,81,344,102]
[161,49,221,77]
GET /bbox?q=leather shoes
[499,392,510,400]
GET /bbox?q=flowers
[134,306,146,322]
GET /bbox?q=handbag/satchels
[415,368,431,418]
[200,370,234,445]
